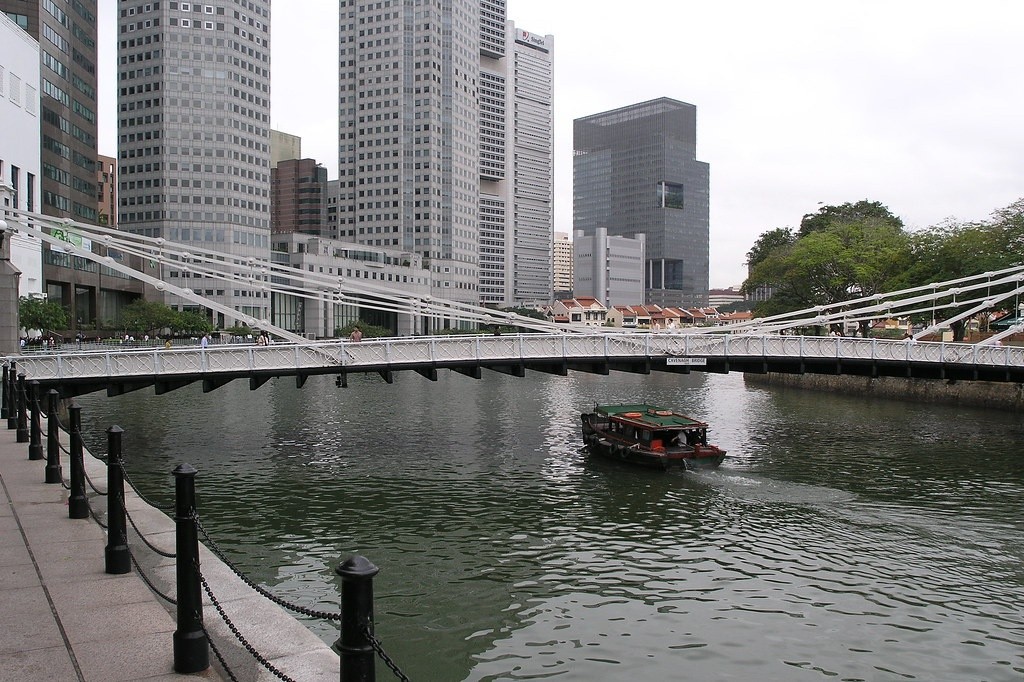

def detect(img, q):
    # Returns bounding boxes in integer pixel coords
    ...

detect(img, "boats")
[580,403,729,471]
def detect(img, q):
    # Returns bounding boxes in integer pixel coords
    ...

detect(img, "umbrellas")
[993,317,1024,327]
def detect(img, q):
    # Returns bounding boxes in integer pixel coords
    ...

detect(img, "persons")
[200,333,209,348]
[671,429,687,447]
[666,318,675,333]
[144,334,150,340]
[257,330,269,345]
[21,338,26,346]
[350,326,363,342]
[494,325,501,336]
[165,338,171,349]
[125,334,135,341]
[42,337,48,351]
[829,330,841,336]
[652,319,660,334]
[48,336,54,351]
